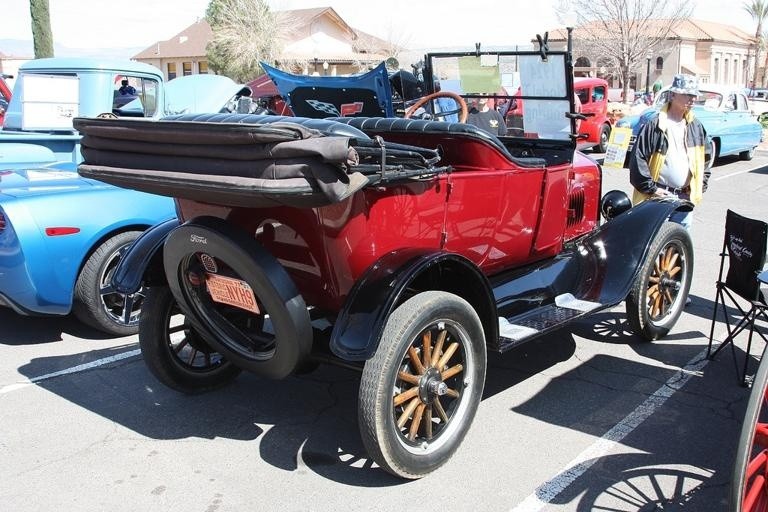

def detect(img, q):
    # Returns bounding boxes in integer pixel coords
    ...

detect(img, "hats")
[666,74,703,99]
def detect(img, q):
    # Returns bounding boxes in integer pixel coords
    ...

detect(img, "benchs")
[324,117,547,170]
[159,114,373,142]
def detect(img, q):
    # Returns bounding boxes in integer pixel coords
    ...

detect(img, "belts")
[655,182,690,195]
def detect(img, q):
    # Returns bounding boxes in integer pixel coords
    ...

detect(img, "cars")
[609,80,765,170]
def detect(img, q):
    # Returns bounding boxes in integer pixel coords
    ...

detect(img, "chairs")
[705,207,768,386]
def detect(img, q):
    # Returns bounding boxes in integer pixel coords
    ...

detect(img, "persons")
[119,80,133,95]
[630,74,713,305]
[653,80,663,98]
[466,86,510,136]
